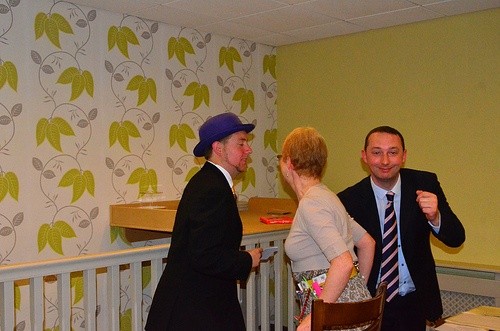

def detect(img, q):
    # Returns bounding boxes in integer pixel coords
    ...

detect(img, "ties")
[380,191,400,303]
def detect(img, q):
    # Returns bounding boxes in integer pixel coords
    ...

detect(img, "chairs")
[311,282,388,331]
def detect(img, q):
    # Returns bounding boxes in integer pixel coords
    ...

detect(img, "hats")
[193,112,255,157]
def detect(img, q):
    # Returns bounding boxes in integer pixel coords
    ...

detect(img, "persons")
[336,126,466,331]
[144,111,264,331]
[275,127,375,331]
